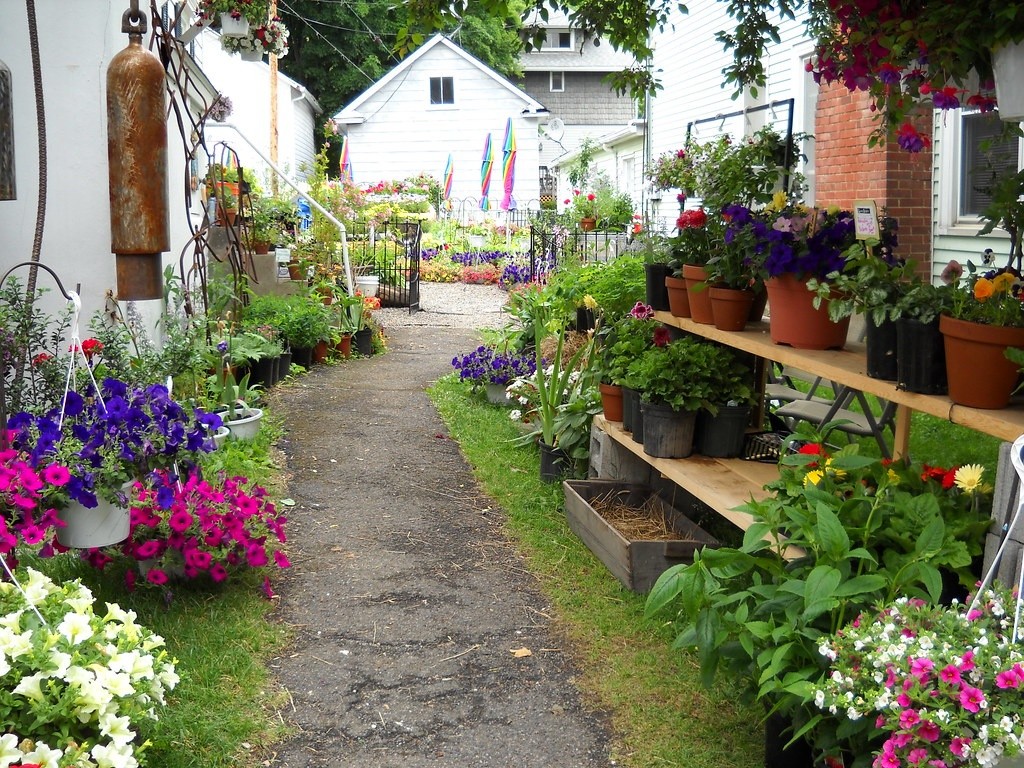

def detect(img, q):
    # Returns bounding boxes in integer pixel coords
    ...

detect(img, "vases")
[866,309,897,382]
[255,241,271,255]
[940,307,1024,409]
[356,274,380,296]
[600,384,622,421]
[641,399,698,458]
[201,425,230,450]
[53,476,136,548]
[683,262,724,325]
[709,283,755,332]
[763,701,816,768]
[240,47,262,61]
[287,261,310,280]
[621,385,632,431]
[336,333,352,359]
[220,12,249,37]
[763,273,856,351]
[580,219,595,231]
[218,408,263,441]
[272,357,278,383]
[138,559,201,582]
[211,389,260,413]
[218,209,237,228]
[749,288,768,324]
[751,165,795,194]
[217,181,239,197]
[485,384,512,403]
[355,329,372,355]
[896,311,947,394]
[318,288,332,305]
[631,391,644,444]
[244,357,271,388]
[700,403,751,457]
[315,340,328,362]
[644,263,678,311]
[208,366,237,383]
[665,276,691,318]
[292,345,313,368]
[280,353,293,377]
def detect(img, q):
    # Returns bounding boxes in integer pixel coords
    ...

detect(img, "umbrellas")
[444,154,453,199]
[499,117,518,212]
[479,133,494,214]
[339,136,354,182]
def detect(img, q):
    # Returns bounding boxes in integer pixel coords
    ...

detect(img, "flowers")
[805,1,1024,155]
[452,344,584,426]
[584,294,764,417]
[191,0,641,308]
[644,124,1024,397]
[0,262,392,768]
[641,419,1024,768]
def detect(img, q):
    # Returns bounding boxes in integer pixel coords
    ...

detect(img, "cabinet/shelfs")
[593,309,1024,563]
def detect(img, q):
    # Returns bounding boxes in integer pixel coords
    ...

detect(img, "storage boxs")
[562,479,719,595]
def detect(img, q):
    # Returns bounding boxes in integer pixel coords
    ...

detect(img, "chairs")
[765,359,911,470]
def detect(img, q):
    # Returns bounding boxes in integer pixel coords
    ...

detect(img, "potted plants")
[505,310,595,484]
